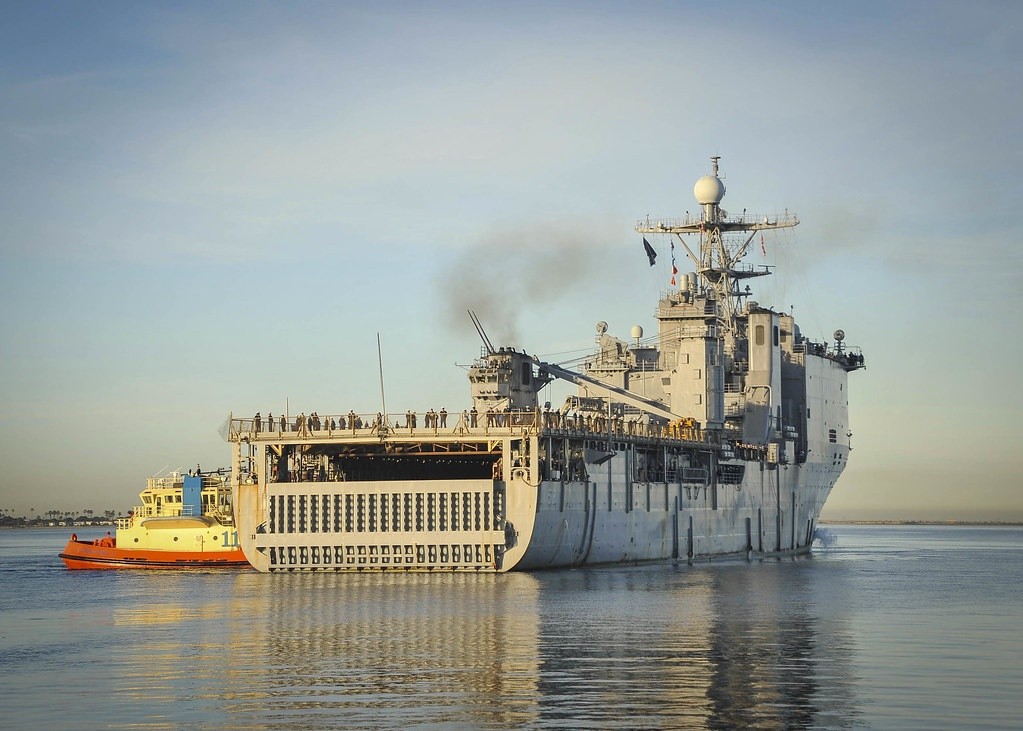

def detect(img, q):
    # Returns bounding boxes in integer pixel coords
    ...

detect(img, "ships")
[223,153,867,575]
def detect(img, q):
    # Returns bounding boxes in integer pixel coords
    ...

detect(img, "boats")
[57,462,253,571]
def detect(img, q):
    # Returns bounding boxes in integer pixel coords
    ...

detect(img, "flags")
[643,237,657,267]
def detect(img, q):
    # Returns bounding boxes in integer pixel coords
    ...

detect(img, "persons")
[544,407,670,436]
[254,406,542,433]
